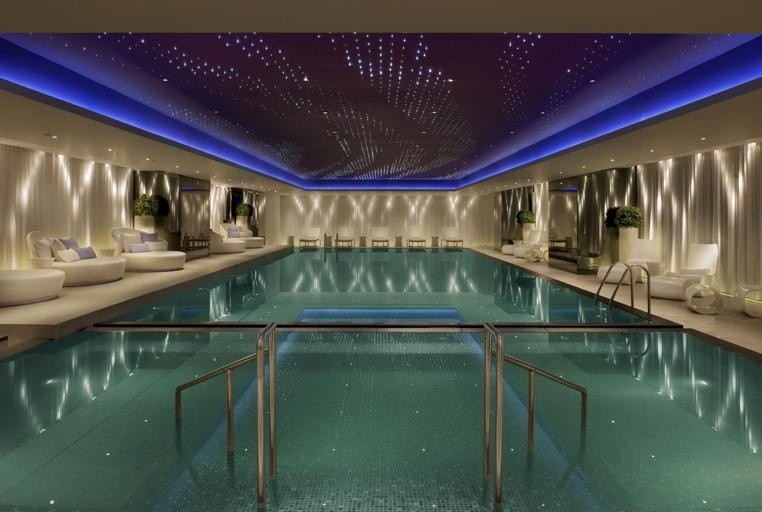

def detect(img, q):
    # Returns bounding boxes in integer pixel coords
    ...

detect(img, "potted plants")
[133,195,160,234]
[515,211,536,241]
[614,205,642,262]
[236,203,250,225]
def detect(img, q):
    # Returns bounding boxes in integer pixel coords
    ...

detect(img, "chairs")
[298,226,465,251]
[502,230,720,303]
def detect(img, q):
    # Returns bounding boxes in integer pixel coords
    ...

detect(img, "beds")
[208,225,244,255]
[111,225,186,272]
[0,268,65,307]
[26,230,126,286]
[219,223,264,249]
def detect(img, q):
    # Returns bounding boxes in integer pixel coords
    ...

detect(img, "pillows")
[123,229,164,253]
[34,236,98,263]
[225,225,247,237]
